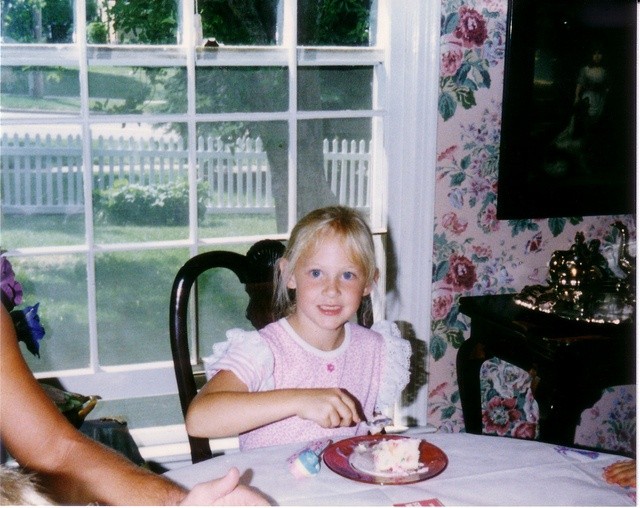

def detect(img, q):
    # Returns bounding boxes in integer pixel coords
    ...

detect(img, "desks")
[458,289,636,447]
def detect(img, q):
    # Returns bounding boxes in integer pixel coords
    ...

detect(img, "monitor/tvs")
[495,0,637,221]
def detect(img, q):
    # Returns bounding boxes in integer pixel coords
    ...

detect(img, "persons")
[0,299,273,507]
[604,460,635,489]
[186,206,412,452]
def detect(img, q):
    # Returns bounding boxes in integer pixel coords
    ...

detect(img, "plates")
[323,435,448,485]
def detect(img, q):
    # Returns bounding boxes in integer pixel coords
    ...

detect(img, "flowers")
[1,251,48,360]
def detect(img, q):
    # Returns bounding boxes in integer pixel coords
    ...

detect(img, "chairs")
[168,238,379,458]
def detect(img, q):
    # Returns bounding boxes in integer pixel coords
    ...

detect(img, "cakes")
[355,438,424,474]
